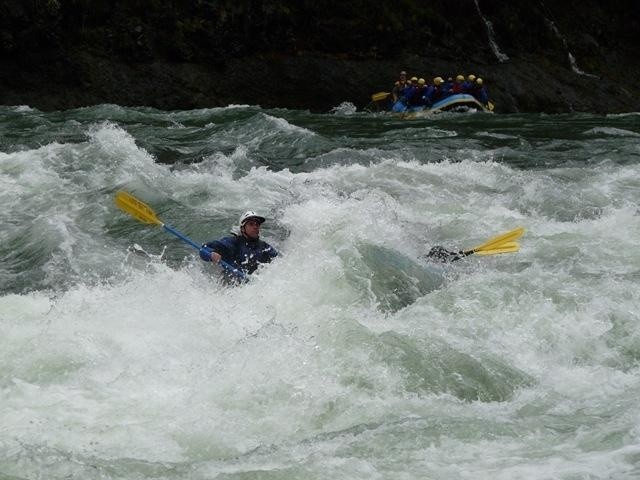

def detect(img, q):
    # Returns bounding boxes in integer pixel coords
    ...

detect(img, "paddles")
[449,227,525,262]
[116,191,250,280]
[372,91,392,101]
[451,241,520,255]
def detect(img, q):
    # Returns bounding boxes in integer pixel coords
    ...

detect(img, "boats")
[364,91,489,116]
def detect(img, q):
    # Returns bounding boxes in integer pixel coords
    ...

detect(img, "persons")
[199,211,278,286]
[392,70,488,113]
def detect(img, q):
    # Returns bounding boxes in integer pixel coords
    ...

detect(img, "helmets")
[400,71,407,75]
[456,75,464,81]
[476,78,483,85]
[239,210,265,229]
[434,77,445,86]
[418,78,425,85]
[410,77,418,82]
[468,74,476,82]
